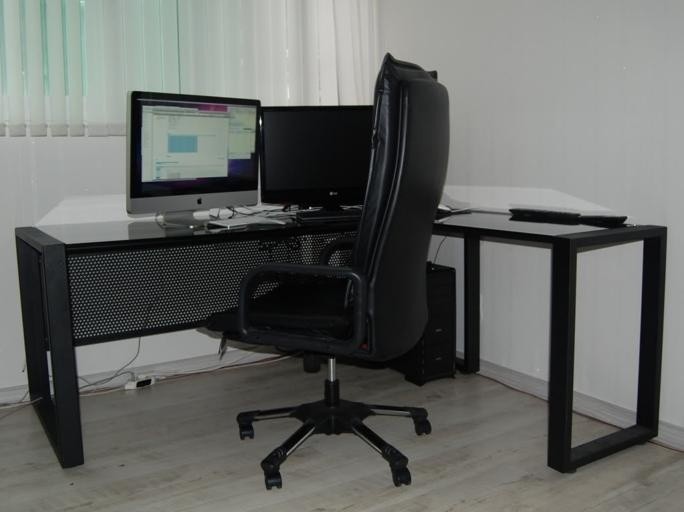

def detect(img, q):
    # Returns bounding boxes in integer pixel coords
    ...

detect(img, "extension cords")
[124,377,155,390]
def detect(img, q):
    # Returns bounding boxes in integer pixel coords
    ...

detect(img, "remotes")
[509,206,580,220]
[579,216,628,227]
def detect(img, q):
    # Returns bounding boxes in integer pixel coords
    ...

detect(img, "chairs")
[204,51,452,489]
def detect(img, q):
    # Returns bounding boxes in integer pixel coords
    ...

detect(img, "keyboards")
[296,210,360,220]
[208,216,265,228]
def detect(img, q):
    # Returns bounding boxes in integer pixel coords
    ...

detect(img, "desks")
[14,201,669,474]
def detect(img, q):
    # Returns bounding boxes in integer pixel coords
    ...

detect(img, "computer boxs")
[389,261,457,387]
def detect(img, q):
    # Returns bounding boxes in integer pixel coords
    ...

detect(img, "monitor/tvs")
[260,105,375,221]
[126,90,262,229]
